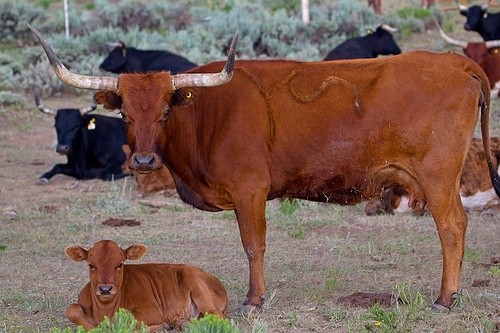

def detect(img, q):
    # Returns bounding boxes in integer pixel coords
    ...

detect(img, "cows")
[431,16,500,98]
[454,0,500,41]
[98,39,199,75]
[26,21,500,315]
[322,23,402,62]
[33,93,176,199]
[64,239,230,333]
[365,136,500,214]
